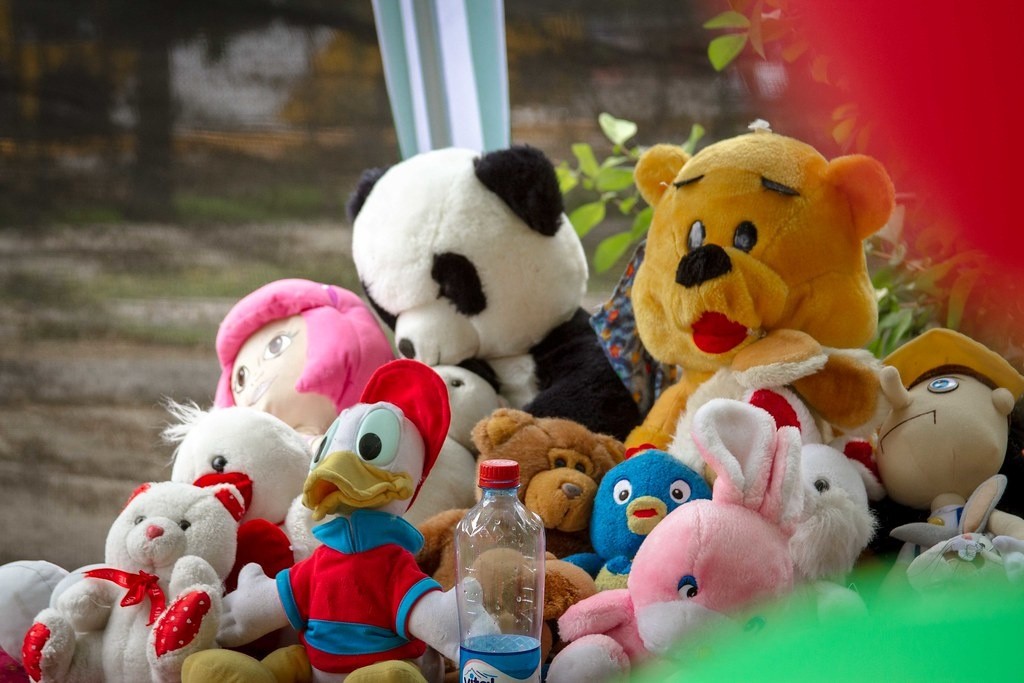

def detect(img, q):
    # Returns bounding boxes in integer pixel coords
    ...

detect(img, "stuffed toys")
[0,329,1024,683]
[349,145,640,439]
[217,280,399,439]
[625,122,893,449]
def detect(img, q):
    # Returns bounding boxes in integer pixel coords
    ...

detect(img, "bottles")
[454,459,546,683]
